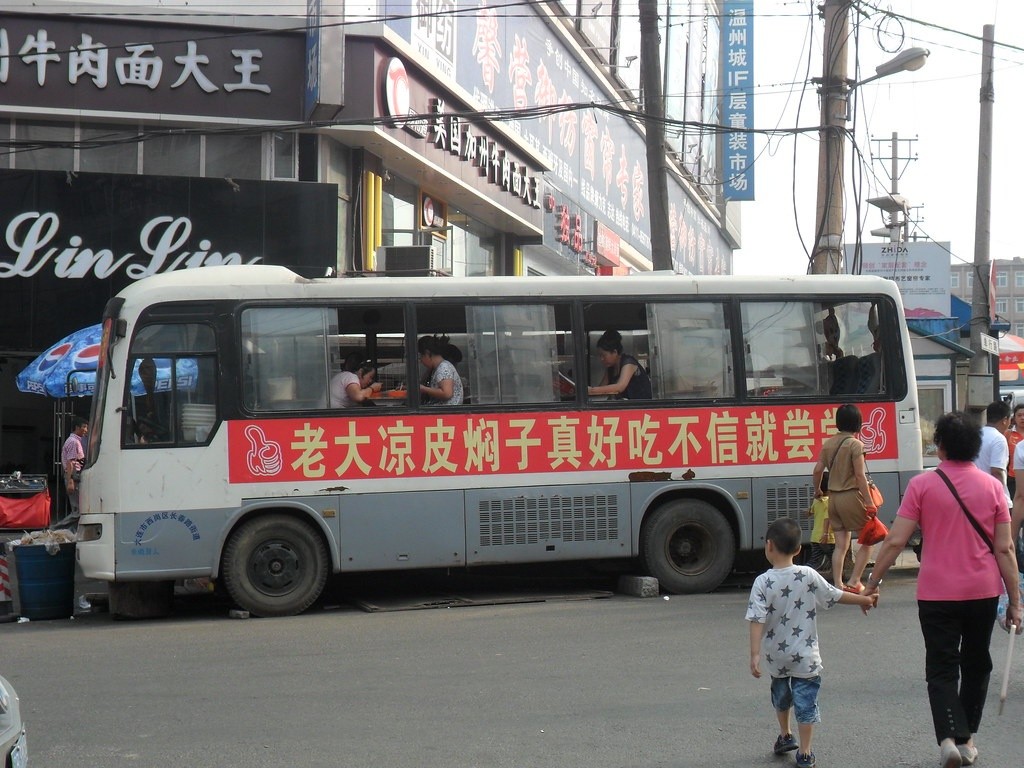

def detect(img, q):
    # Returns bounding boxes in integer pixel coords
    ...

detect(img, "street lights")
[865,191,910,242]
[811,46,928,274]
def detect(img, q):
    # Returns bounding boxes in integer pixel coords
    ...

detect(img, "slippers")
[841,583,864,594]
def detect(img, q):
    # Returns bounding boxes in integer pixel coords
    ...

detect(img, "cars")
[1000,389,1024,418]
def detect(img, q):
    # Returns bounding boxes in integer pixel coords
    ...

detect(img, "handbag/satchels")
[858,478,884,509]
[856,506,890,546]
[997,573,1024,635]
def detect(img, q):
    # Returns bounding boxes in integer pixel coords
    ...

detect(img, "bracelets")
[866,573,883,587]
[591,385,595,394]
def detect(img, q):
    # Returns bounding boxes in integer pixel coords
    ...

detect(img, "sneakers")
[796,748,816,768]
[773,733,799,755]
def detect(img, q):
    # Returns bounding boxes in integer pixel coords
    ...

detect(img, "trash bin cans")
[8,528,78,622]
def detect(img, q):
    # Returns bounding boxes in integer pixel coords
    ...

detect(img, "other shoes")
[955,744,977,765]
[940,741,962,768]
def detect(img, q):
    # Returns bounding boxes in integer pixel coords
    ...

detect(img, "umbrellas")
[999,332,1024,381]
[15,322,267,445]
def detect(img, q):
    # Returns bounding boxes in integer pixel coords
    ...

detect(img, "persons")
[50,416,88,531]
[859,410,1024,768]
[315,325,653,408]
[812,404,879,597]
[745,518,879,768]
[976,400,1024,576]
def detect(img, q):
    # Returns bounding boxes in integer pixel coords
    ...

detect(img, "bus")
[64,264,925,619]
